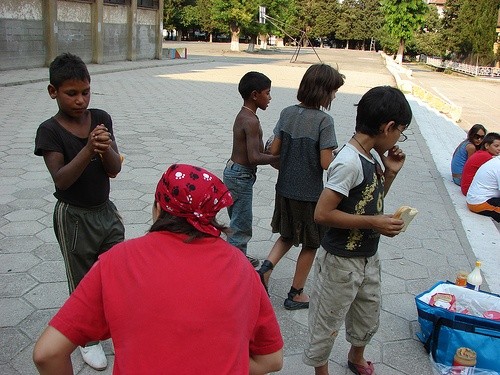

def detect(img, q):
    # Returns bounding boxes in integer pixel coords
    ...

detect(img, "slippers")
[347,359,377,375]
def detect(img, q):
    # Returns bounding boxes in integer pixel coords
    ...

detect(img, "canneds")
[453,347,477,367]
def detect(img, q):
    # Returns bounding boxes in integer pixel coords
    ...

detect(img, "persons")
[300,86,413,375]
[451,123,487,186]
[466,154,500,223]
[32,165,284,375]
[34,52,126,370]
[222,72,281,265]
[461,133,500,195]
[253,63,345,307]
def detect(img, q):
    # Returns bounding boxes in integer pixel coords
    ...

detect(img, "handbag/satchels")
[413,278,500,374]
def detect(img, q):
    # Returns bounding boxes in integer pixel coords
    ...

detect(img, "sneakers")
[247,255,257,265]
[78,341,108,371]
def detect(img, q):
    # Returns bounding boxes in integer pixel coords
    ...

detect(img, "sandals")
[284,286,311,310]
[255,260,275,298]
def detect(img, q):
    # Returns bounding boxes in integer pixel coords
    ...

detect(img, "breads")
[393,206,418,232]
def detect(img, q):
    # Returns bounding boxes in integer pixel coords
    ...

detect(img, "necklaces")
[352,134,386,177]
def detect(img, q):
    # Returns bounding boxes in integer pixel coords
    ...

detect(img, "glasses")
[393,125,408,143]
[473,133,485,140]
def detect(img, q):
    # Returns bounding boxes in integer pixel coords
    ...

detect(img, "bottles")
[466,261,482,291]
